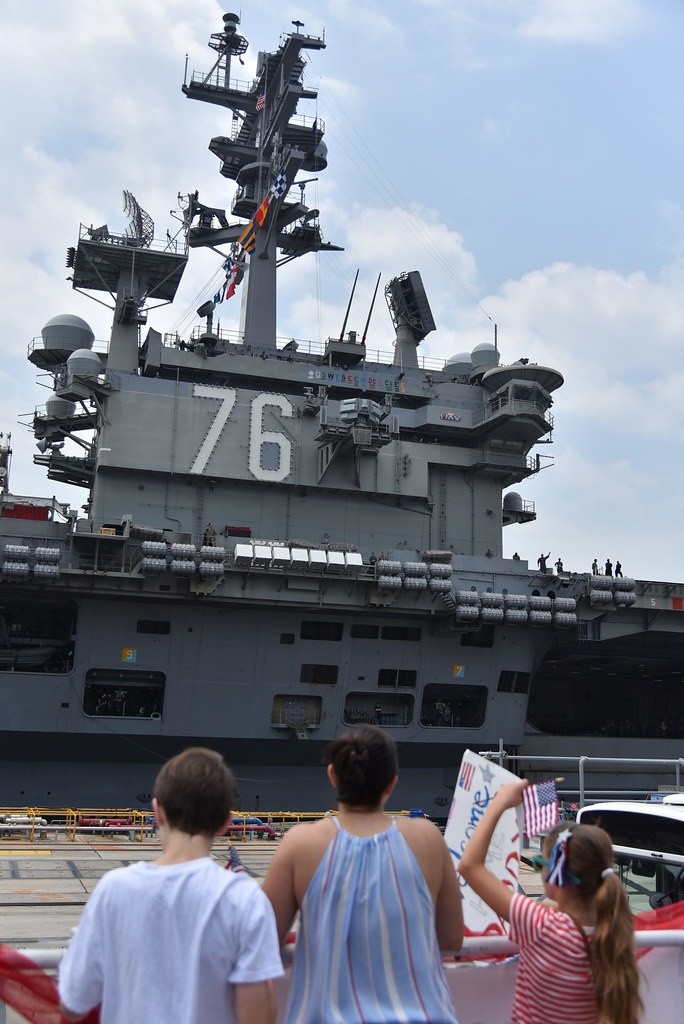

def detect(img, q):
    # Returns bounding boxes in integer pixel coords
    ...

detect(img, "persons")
[260,725,467,1023]
[180,340,186,350]
[59,749,288,1024]
[537,552,551,574]
[457,780,647,1024]
[485,549,493,557]
[555,557,563,574]
[615,561,623,577]
[605,559,613,576]
[592,558,600,576]
[513,552,520,560]
[203,522,218,546]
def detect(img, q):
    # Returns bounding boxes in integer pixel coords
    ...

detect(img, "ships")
[1,10,684,808]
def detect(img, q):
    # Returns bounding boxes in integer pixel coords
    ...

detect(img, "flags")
[443,749,522,964]
[254,168,293,228]
[520,781,558,840]
[238,223,256,255]
[213,257,241,304]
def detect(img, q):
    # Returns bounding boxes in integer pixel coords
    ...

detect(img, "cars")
[575,792,684,869]
